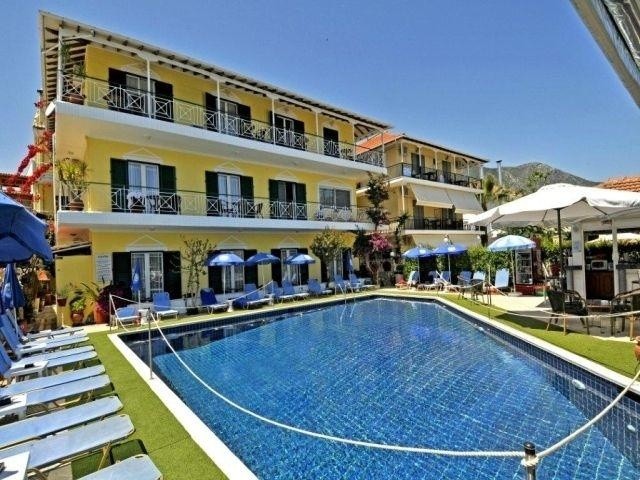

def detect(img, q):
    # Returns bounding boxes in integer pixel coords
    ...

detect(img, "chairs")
[249,203,264,219]
[130,196,145,213]
[545,285,592,335]
[0,292,178,480]
[396,269,510,293]
[163,195,181,215]
[423,219,433,229]
[219,199,237,217]
[199,273,376,313]
[610,287,640,341]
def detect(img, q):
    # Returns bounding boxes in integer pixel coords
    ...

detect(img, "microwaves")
[591,260,608,270]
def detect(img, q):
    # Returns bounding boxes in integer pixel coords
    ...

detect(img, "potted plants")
[394,265,404,283]
[66,61,89,105]
[309,225,348,294]
[103,95,123,111]
[80,277,114,323]
[56,288,68,306]
[69,280,87,326]
[54,156,94,211]
[169,238,218,315]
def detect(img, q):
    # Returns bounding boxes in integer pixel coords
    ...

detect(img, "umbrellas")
[0,191,57,268]
[206,252,245,294]
[0,263,28,339]
[284,252,316,285]
[241,252,281,293]
[130,258,145,308]
[465,182,640,291]
[482,234,537,292]
[402,245,434,284]
[431,242,468,283]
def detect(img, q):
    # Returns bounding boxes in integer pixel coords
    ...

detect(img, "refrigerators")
[514,237,541,295]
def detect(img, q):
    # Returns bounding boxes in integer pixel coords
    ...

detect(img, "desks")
[455,180,467,185]
[146,195,160,213]
[587,300,614,335]
